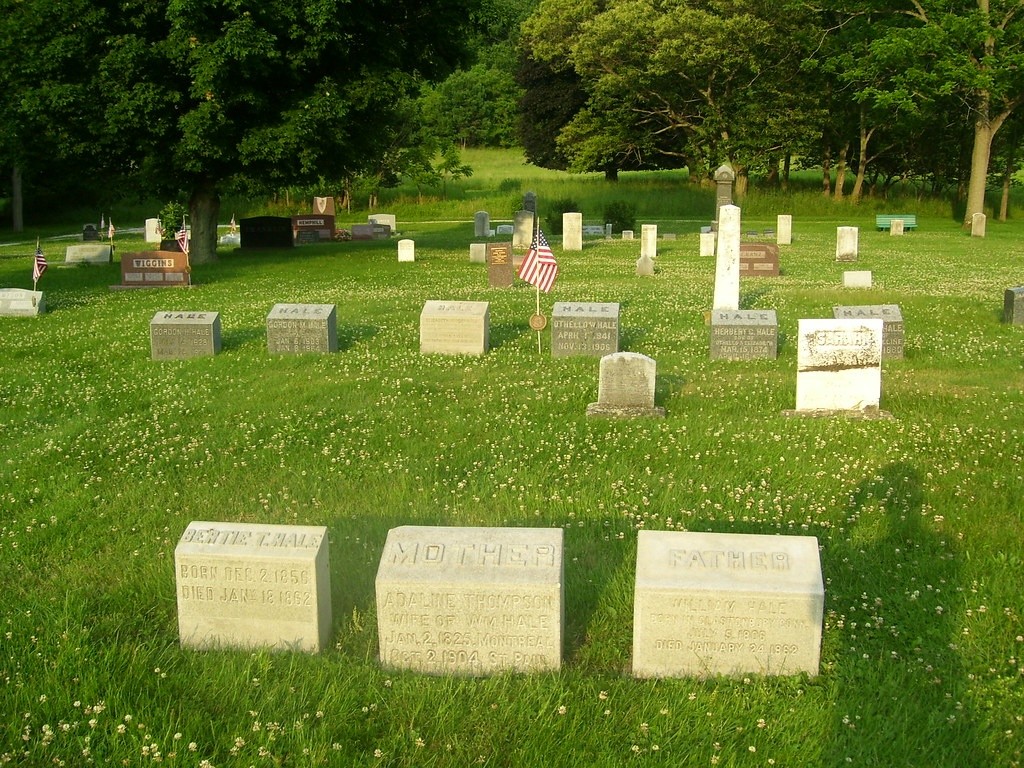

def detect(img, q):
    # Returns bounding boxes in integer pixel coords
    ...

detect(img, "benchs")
[876,215,918,232]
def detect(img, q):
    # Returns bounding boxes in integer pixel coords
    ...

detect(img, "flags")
[100,217,105,228]
[32,248,47,282]
[518,229,558,293]
[108,224,115,238]
[231,218,236,231]
[155,223,161,235]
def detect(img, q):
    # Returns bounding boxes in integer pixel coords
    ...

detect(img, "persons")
[176,226,189,254]
